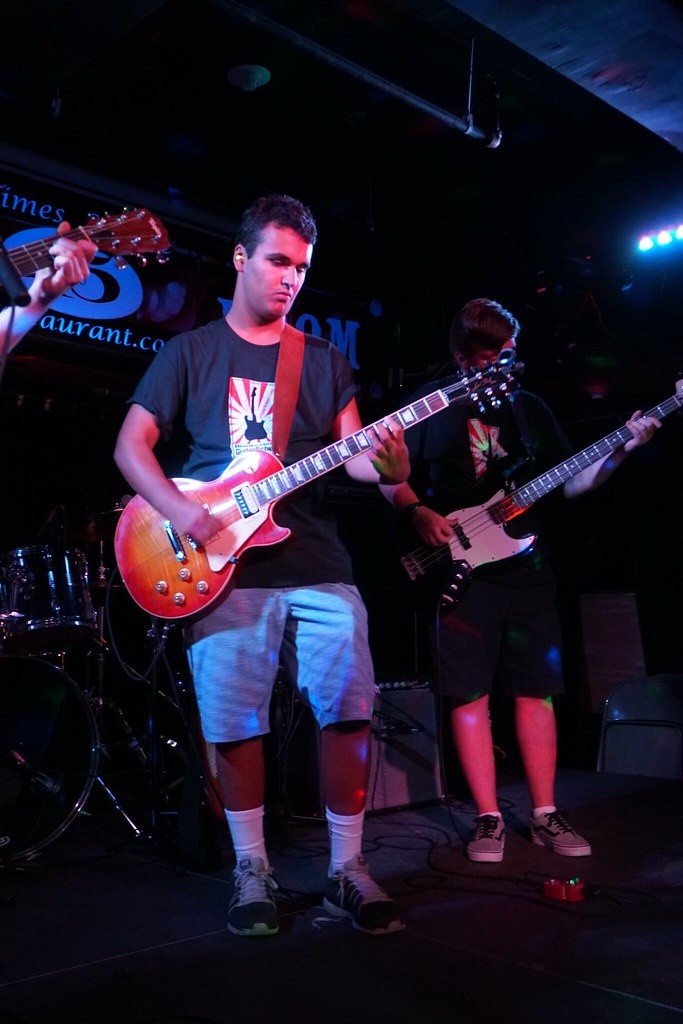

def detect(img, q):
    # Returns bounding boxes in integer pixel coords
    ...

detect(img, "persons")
[377,296,662,863]
[113,201,412,939]
[0,217,100,370]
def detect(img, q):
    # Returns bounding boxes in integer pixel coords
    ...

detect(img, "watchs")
[406,502,423,520]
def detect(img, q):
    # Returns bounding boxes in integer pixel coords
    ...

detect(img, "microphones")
[39,504,63,536]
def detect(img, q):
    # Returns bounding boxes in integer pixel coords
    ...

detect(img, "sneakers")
[225,857,281,935]
[468,814,505,862]
[530,810,591,857]
[313,856,407,935]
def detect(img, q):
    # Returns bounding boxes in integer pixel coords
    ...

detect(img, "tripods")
[69,502,209,845]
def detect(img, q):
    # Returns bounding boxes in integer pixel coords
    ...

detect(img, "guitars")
[399,376,683,617]
[0,204,171,287]
[114,359,521,623]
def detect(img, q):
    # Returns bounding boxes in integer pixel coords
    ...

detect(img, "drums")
[0,544,98,657]
[0,650,102,866]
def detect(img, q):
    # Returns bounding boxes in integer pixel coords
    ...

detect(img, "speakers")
[313,676,448,818]
[323,475,420,684]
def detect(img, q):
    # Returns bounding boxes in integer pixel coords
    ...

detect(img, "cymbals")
[96,506,123,515]
[65,582,125,590]
